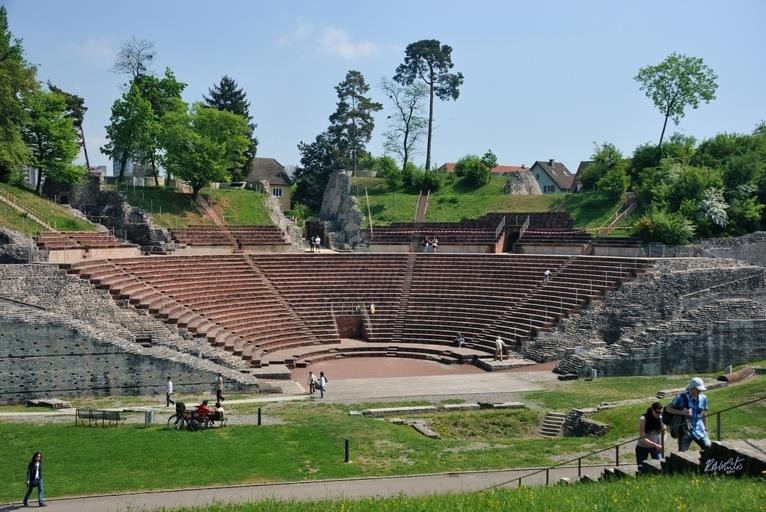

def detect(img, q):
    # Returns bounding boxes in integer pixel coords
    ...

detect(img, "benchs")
[74,407,125,428]
[183,410,227,429]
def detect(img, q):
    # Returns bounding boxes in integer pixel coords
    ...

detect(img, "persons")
[317,370,328,399]
[214,372,225,403]
[197,400,214,428]
[163,376,176,408]
[492,335,505,362]
[308,235,314,252]
[452,332,460,346]
[311,234,321,253]
[368,301,375,315]
[665,375,714,453]
[22,450,49,507]
[457,333,465,349]
[211,402,225,426]
[428,235,439,253]
[630,401,668,466]
[544,268,552,284]
[420,235,431,253]
[307,370,318,396]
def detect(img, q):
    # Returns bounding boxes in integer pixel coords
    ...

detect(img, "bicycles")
[168,409,207,431]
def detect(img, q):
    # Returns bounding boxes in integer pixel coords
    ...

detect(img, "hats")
[692,377,706,390]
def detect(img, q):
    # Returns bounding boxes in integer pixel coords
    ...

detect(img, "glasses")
[654,409,663,414]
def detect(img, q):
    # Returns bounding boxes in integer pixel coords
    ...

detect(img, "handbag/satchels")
[662,393,690,430]
[30,481,39,486]
